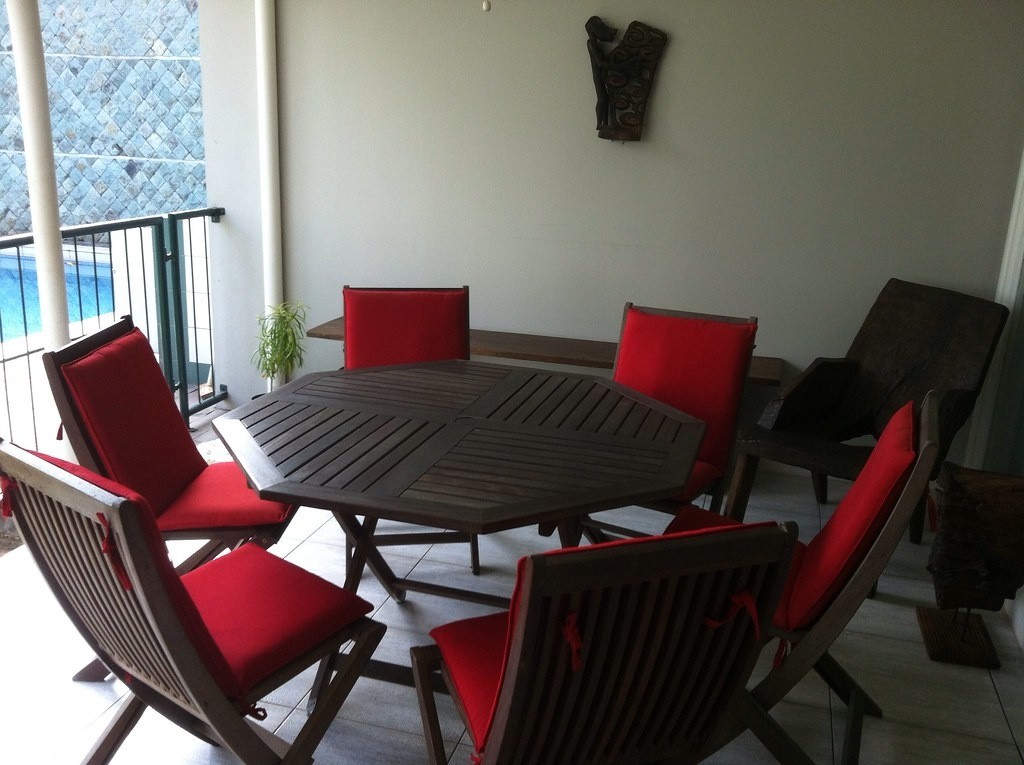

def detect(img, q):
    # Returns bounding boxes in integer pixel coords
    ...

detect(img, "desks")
[305,316,787,491]
[210,359,706,717]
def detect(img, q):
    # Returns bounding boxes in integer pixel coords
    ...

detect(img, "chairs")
[43,315,299,555]
[0,438,389,765]
[723,275,1011,546]
[410,520,799,765]
[584,304,760,542]
[665,389,941,764]
[341,287,481,576]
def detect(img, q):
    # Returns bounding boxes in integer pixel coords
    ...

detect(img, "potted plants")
[251,300,310,402]
